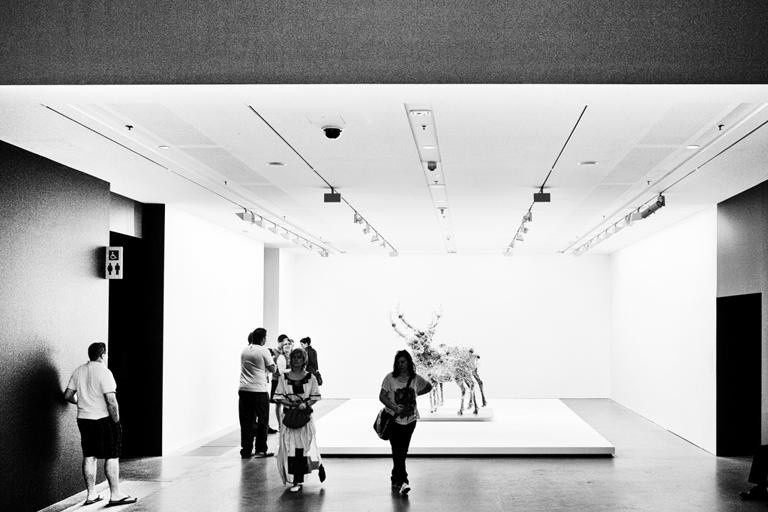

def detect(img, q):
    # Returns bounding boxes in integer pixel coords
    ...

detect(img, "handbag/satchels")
[282,407,310,429]
[373,408,395,440]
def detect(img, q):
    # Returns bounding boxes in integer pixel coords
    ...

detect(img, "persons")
[238,328,326,492]
[64,343,137,505]
[380,350,432,494]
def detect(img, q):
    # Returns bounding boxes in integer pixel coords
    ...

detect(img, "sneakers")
[392,481,411,495]
[242,450,275,459]
[268,428,277,434]
[289,484,302,492]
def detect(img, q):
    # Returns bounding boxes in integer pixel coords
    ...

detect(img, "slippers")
[109,495,137,505]
[83,495,103,505]
